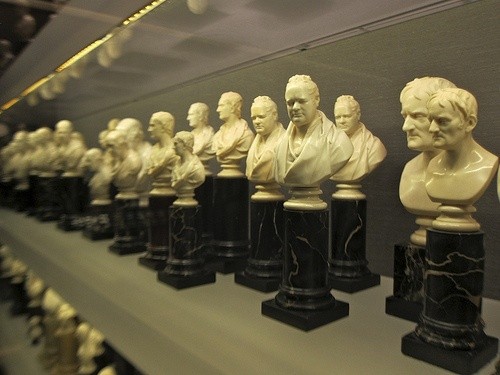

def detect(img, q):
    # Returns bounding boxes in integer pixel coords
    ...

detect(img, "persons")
[211,91,255,164]
[245,95,289,185]
[144,110,176,180]
[399,76,462,218]
[170,131,206,193]
[327,95,388,184]
[424,87,500,207]
[0,118,152,193]
[0,244,117,375]
[186,102,217,163]
[272,74,354,188]
